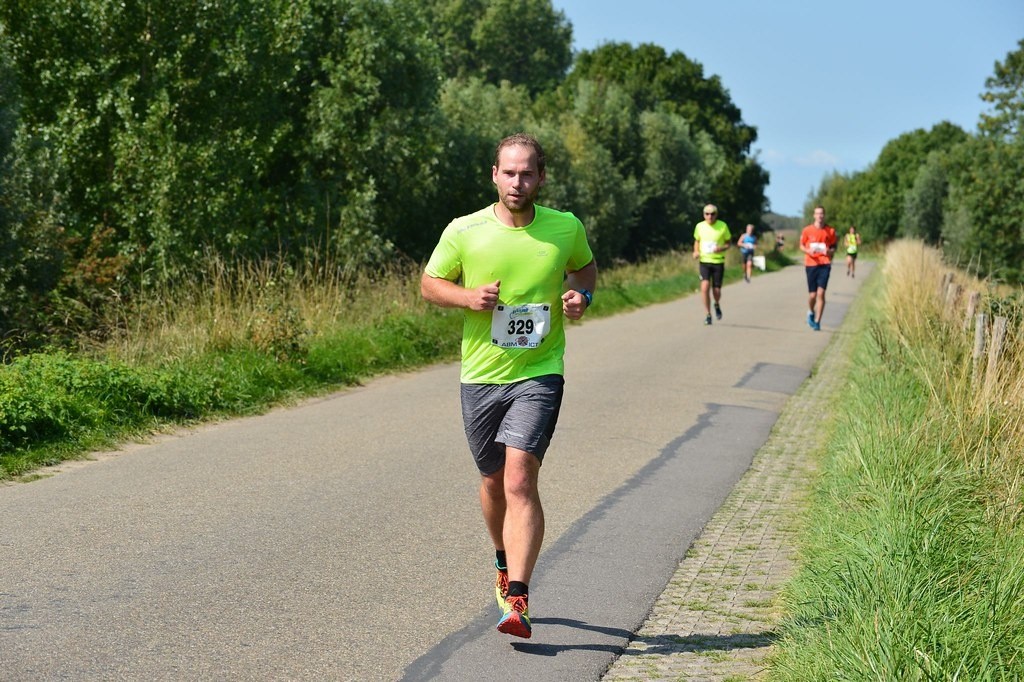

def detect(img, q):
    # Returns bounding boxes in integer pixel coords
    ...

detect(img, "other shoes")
[814,323,820,330]
[808,309,815,327]
[706,317,711,323]
[715,304,721,318]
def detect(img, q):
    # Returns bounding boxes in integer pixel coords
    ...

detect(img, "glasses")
[706,213,714,215]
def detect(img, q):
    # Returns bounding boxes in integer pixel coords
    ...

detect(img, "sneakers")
[497,589,531,638]
[495,563,509,616]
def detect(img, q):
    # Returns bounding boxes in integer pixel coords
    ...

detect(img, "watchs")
[579,289,592,307]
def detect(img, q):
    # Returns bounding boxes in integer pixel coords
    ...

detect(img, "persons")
[737,224,759,283]
[776,233,784,252]
[692,204,732,325]
[844,226,861,279]
[421,133,598,638]
[800,205,837,331]
